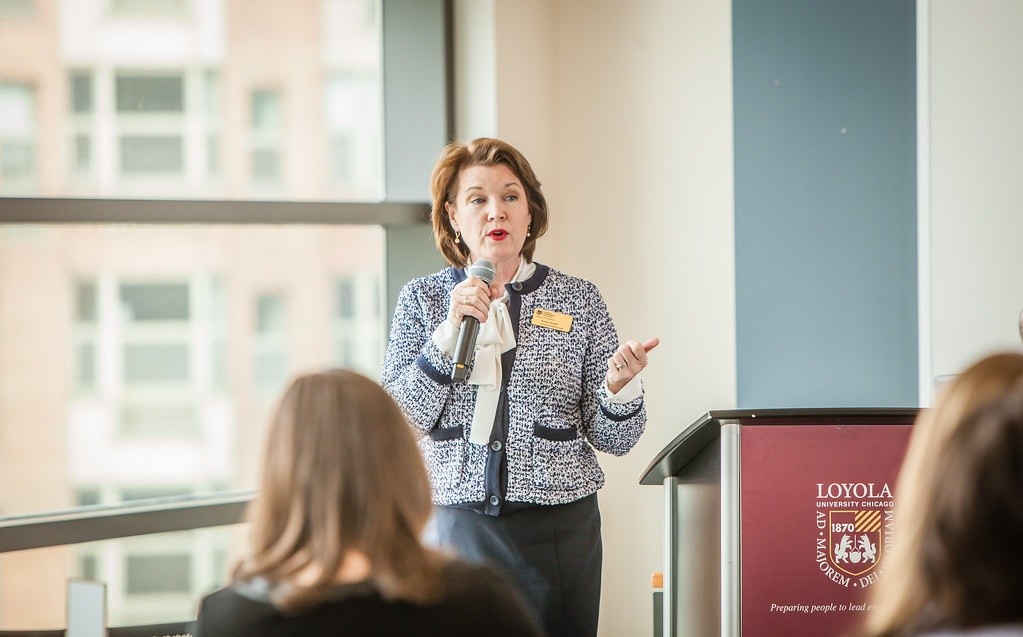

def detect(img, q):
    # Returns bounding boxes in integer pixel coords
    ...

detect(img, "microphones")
[450,260,495,381]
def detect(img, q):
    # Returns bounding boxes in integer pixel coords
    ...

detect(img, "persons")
[381,135,660,637]
[855,351,1023,636]
[191,367,543,636]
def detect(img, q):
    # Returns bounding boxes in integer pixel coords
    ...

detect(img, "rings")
[616,361,627,370]
[463,296,470,306]
